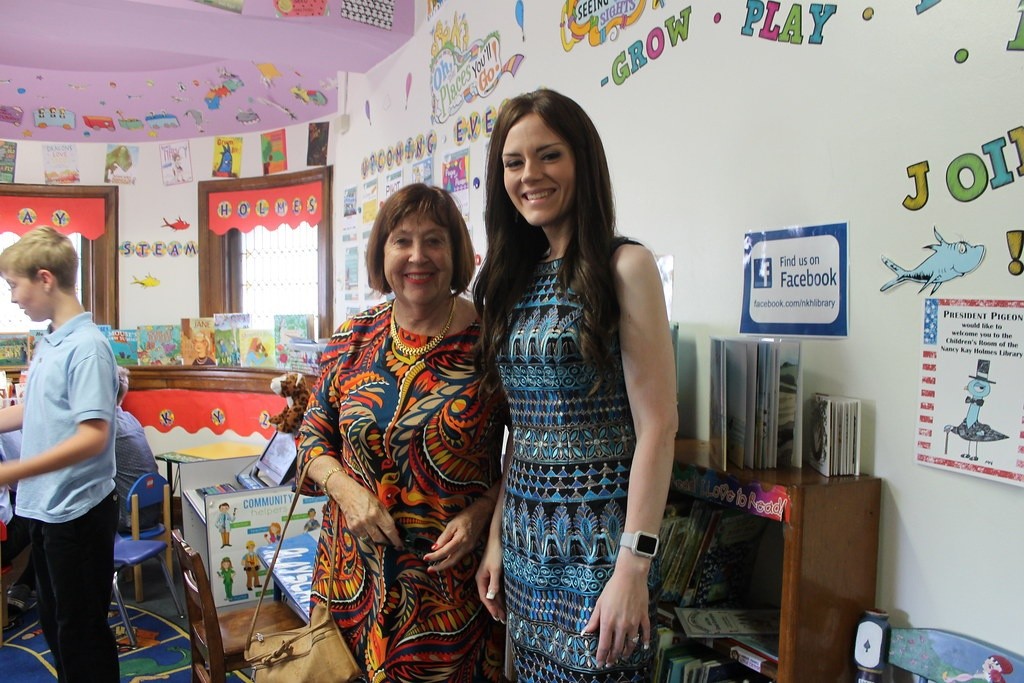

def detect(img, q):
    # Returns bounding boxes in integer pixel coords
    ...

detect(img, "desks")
[257,533,317,625]
[155,452,205,509]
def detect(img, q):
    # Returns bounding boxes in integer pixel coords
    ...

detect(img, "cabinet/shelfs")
[180,455,330,607]
[657,438,880,683]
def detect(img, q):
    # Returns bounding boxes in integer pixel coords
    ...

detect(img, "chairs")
[853,608,1024,683]
[119,473,173,603]
[172,529,307,683]
[110,532,185,649]
[0,520,13,626]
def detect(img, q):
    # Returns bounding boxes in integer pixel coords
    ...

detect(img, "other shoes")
[7,581,28,610]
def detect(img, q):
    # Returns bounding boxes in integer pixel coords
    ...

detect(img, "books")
[652,500,780,683]
[670,321,861,476]
[1,312,332,410]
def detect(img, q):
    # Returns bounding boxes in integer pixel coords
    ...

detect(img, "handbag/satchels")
[244,603,362,683]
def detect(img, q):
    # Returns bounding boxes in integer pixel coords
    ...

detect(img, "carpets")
[0,598,253,683]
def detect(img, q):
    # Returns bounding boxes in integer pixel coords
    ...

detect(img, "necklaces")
[391,296,457,355]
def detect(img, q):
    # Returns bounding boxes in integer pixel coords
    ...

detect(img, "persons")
[0,365,161,631]
[291,184,514,683]
[472,88,678,683]
[0,226,120,683]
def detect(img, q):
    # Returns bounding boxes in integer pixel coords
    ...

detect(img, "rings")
[359,535,370,543]
[627,634,639,646]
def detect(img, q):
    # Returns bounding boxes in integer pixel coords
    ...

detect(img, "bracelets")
[322,467,346,500]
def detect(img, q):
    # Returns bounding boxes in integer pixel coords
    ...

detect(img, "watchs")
[620,531,660,559]
